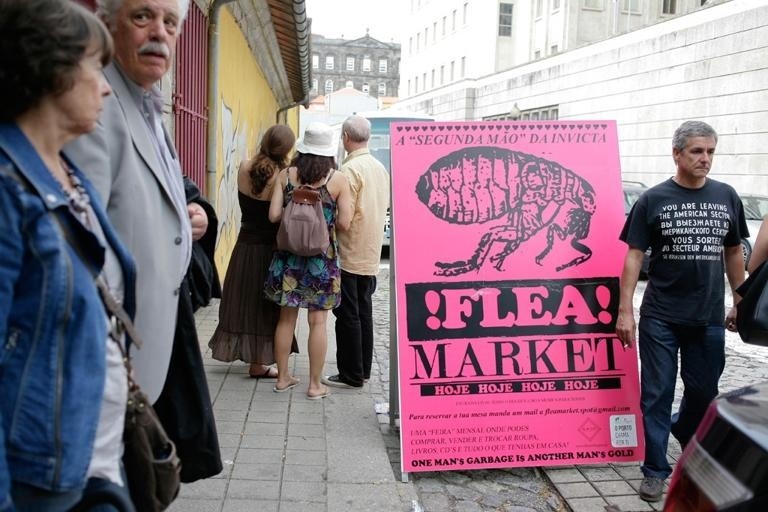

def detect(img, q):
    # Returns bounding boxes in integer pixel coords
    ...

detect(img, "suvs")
[621,178,650,275]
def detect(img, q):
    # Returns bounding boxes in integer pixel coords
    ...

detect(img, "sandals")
[249,365,280,379]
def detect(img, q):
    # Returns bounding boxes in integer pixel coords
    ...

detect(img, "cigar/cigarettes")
[623,344,630,348]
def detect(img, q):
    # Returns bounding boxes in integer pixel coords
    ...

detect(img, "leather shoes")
[640,475,666,502]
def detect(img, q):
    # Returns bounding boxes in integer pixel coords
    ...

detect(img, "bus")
[336,111,440,256]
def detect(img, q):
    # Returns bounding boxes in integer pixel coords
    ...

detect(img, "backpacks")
[274,183,332,255]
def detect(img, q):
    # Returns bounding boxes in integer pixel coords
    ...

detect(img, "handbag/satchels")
[126,379,181,511]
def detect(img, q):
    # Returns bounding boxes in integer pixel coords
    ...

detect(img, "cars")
[735,193,768,270]
[663,379,768,510]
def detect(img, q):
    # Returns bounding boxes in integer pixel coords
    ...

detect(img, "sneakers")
[322,373,363,390]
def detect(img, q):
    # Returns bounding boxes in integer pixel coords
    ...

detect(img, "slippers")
[272,376,303,393]
[307,386,333,401]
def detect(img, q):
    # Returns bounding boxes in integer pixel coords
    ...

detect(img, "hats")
[294,123,337,158]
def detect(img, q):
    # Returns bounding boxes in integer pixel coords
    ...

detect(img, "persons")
[267,127,355,399]
[747,214,768,277]
[613,120,752,503]
[207,126,298,379]
[2,2,159,511]
[57,2,214,511]
[321,112,391,389]
